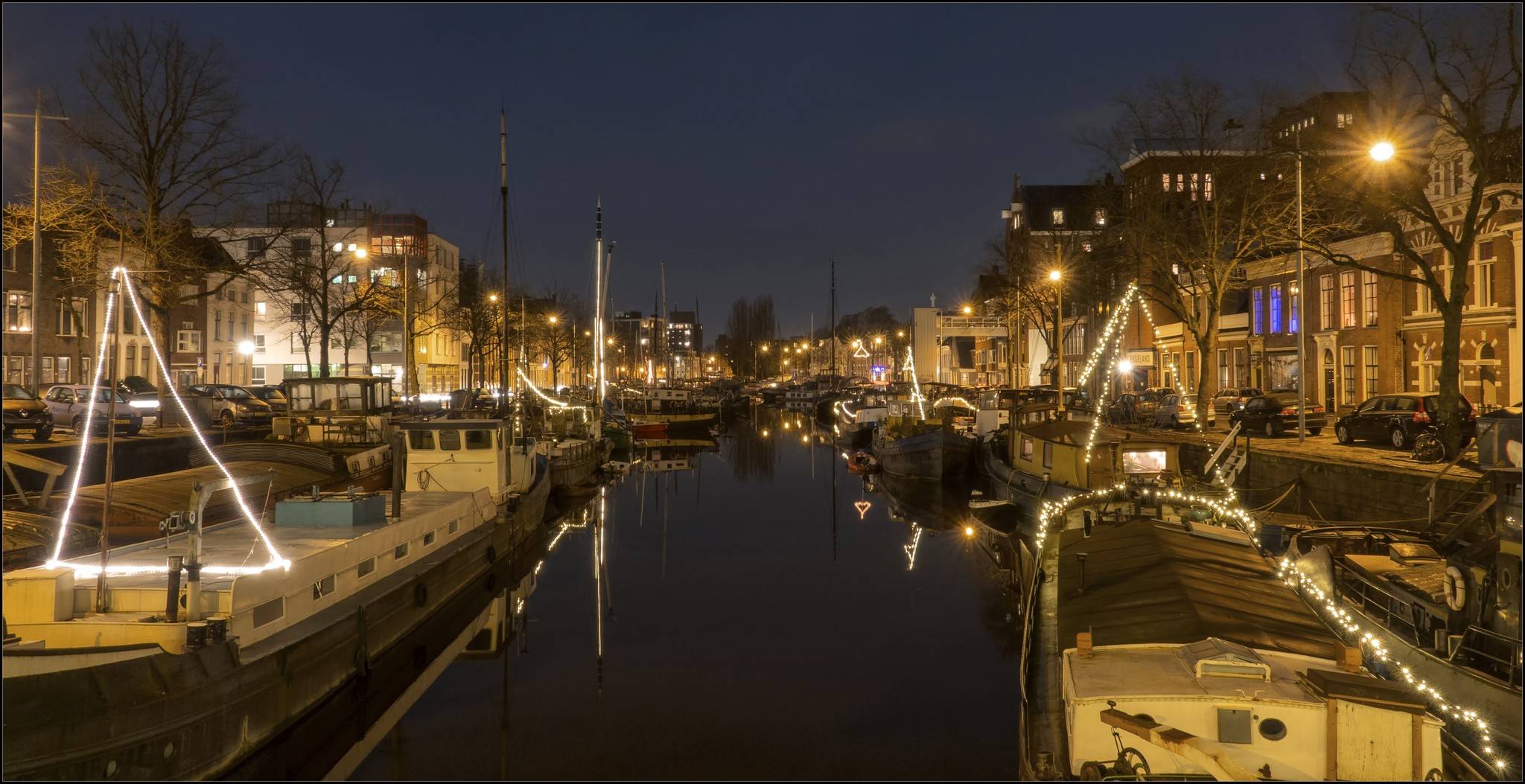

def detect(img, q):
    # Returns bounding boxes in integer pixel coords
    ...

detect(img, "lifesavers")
[884,427,889,436]
[670,400,678,408]
[679,400,690,408]
[1442,566,1466,612]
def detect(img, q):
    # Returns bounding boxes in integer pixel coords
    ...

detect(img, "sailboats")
[0,117,1525,784]
